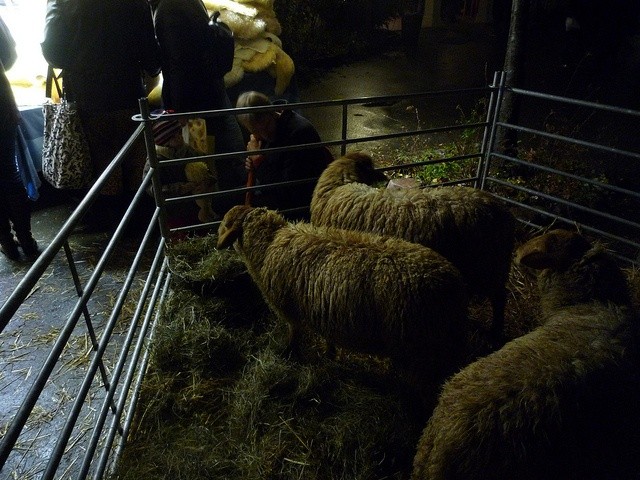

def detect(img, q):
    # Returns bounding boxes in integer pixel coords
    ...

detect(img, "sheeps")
[216,203,496,360]
[308,152,534,259]
[412,228,640,480]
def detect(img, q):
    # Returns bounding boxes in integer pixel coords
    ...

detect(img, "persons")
[233,89,335,214]
[155,1,235,151]
[0,18,42,263]
[144,110,217,234]
[41,0,155,232]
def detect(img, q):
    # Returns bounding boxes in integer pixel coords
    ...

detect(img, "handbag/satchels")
[40,61,93,190]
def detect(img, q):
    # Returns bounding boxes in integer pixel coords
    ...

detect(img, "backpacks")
[196,2,234,76]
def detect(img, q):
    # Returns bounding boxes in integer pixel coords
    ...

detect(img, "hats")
[149,109,185,145]
